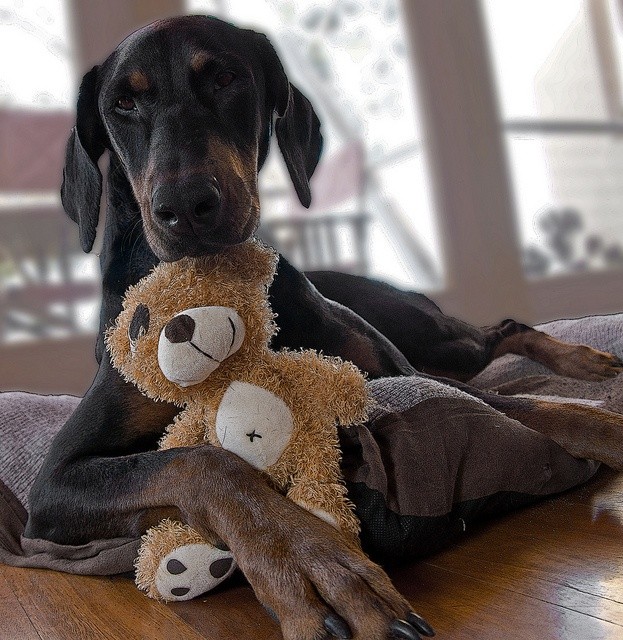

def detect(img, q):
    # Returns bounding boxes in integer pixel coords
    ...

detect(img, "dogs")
[25,14,623,640]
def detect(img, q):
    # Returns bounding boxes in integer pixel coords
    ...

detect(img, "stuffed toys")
[102,236,377,604]
